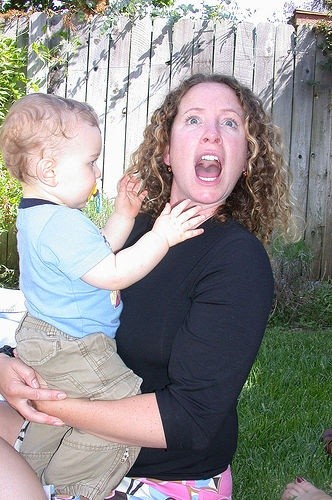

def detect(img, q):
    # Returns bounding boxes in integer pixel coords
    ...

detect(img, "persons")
[0,93,206,500]
[0,73,308,500]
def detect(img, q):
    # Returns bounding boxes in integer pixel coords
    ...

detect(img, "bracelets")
[0,344,17,358]
